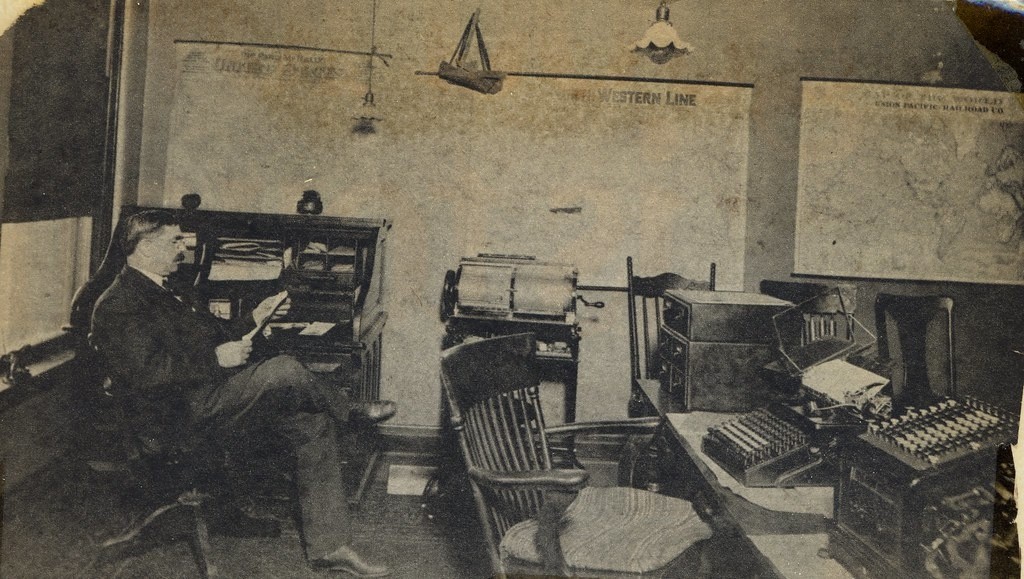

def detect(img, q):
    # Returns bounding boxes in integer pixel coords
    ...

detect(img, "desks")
[661,409,1024,577]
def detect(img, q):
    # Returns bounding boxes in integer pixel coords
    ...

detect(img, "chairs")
[627,256,716,415]
[441,331,717,579]
[874,293,956,408]
[63,326,287,576]
[762,279,857,348]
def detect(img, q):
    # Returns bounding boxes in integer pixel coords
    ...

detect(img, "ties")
[162,280,193,309]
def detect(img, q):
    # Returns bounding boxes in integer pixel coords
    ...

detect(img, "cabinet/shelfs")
[420,250,581,516]
[117,210,392,503]
[654,288,801,410]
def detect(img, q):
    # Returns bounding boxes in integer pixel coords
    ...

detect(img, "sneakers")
[347,399,397,431]
[309,545,390,579]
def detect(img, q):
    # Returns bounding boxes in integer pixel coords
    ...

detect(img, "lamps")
[627,1,694,60]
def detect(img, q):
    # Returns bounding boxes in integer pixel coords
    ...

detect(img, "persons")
[92,205,402,577]
